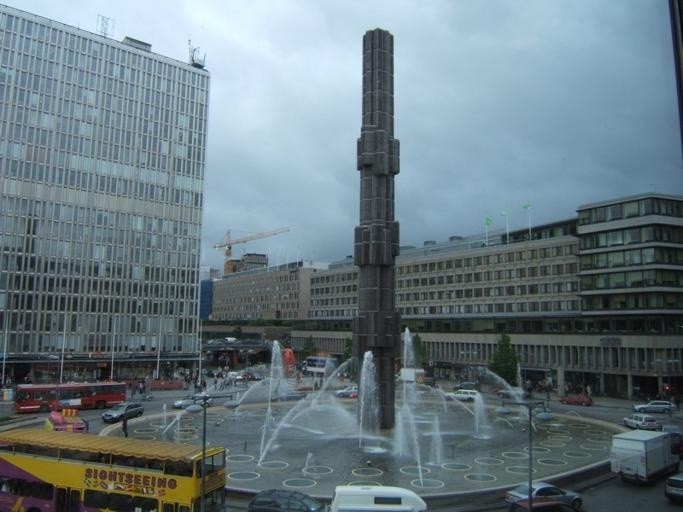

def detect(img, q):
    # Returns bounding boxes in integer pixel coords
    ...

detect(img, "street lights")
[495,398,553,511]
[186,393,239,512]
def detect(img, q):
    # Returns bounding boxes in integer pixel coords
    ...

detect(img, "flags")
[518,202,530,209]
[483,214,492,228]
[499,209,507,215]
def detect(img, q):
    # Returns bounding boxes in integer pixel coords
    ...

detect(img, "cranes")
[212,225,291,274]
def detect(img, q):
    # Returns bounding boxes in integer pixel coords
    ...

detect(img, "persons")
[523,377,602,400]
[0,350,230,395]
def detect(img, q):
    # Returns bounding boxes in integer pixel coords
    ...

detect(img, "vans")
[329,483,427,512]
[446,389,479,401]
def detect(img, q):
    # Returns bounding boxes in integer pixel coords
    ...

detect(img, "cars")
[174,392,211,409]
[498,385,529,400]
[666,474,683,504]
[227,369,263,382]
[504,479,583,512]
[102,402,144,423]
[283,391,305,400]
[660,423,682,445]
[561,392,592,407]
[336,384,357,398]
[208,336,241,344]
[456,383,481,392]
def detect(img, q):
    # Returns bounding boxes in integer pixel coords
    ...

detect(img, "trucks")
[394,367,425,382]
[610,431,680,482]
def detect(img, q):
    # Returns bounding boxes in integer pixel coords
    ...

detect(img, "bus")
[14,382,126,413]
[302,356,338,377]
[0,428,226,511]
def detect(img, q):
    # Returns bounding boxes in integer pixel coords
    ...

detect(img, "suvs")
[634,399,674,413]
[249,487,322,512]
[625,413,658,430]
